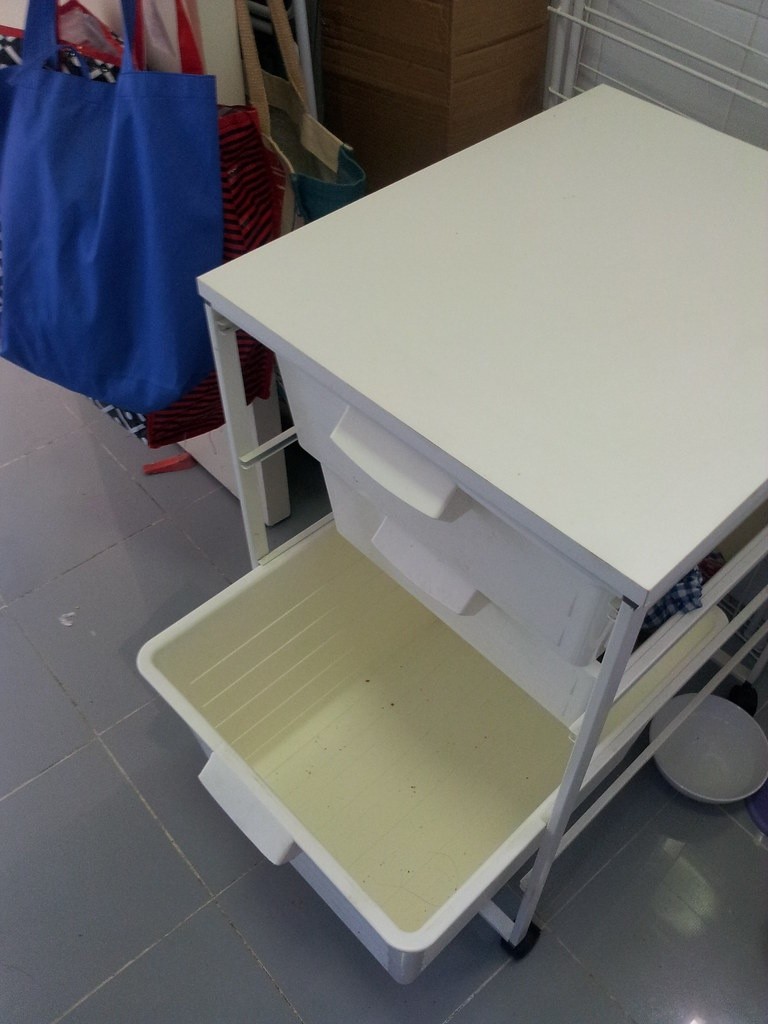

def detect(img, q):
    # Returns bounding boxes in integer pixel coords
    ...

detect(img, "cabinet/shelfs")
[135,82,768,986]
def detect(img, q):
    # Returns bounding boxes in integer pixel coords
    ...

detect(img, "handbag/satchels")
[0,1,370,447]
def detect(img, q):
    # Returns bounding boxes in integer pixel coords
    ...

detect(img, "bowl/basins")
[649,693,768,805]
